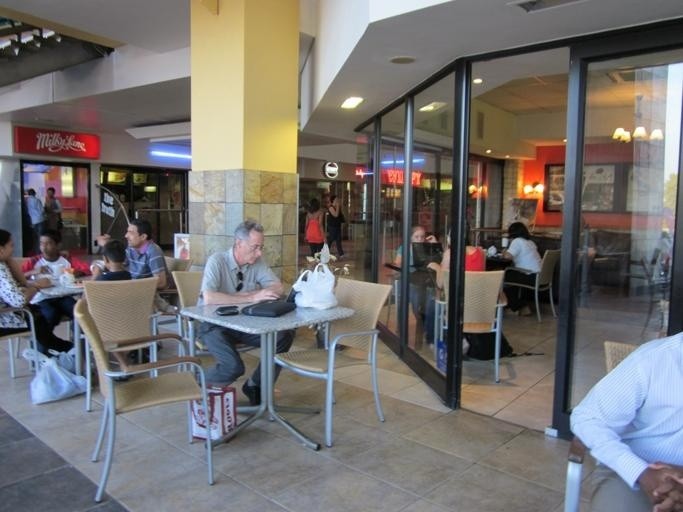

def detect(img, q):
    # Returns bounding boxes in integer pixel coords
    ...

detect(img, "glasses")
[245,244,266,251]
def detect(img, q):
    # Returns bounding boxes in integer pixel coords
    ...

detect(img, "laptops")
[412,242,444,272]
[262,268,307,302]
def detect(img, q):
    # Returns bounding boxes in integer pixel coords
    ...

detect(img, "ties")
[236,271,244,292]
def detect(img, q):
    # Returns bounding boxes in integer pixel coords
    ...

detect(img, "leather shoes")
[241,378,262,407]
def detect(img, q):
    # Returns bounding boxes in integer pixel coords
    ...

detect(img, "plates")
[33,266,90,289]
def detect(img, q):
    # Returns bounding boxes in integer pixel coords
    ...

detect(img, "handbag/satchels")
[190,381,237,444]
[241,298,297,318]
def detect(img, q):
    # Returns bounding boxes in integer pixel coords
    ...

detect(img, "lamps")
[609,94,664,144]
[521,182,545,193]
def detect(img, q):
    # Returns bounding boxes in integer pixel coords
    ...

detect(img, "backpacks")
[468,321,513,361]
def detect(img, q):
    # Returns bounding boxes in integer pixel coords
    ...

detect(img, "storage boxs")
[187,386,238,441]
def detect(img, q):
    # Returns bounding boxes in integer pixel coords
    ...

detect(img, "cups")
[483,245,504,258]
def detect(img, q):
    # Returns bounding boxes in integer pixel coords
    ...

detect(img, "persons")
[429,224,488,356]
[326,195,345,258]
[0,187,171,381]
[305,198,328,256]
[195,222,293,406]
[501,222,542,317]
[396,225,436,267]
[568,327,683,512]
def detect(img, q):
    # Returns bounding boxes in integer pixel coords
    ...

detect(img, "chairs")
[593,230,629,293]
[385,278,400,334]
[639,257,671,334]
[0,256,192,411]
[437,270,507,382]
[577,247,589,308]
[174,269,257,378]
[74,299,216,503]
[504,249,560,323]
[271,276,393,448]
[561,340,643,512]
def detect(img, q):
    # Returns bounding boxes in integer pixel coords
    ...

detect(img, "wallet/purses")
[216,305,239,316]
